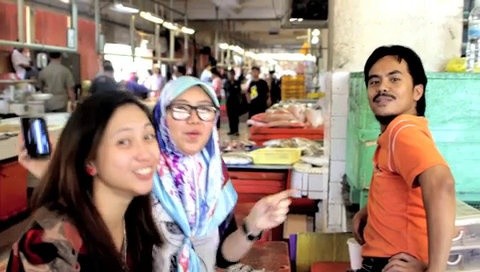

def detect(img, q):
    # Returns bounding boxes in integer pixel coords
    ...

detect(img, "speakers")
[290,0,328,21]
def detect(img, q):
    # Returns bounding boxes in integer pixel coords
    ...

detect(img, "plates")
[33,94,53,100]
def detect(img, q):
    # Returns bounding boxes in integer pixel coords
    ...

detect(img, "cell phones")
[21,117,51,158]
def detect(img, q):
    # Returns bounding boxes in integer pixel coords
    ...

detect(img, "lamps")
[109,0,245,55]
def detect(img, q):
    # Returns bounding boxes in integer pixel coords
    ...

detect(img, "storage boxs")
[446,199,480,272]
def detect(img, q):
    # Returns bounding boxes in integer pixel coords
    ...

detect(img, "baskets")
[250,148,302,164]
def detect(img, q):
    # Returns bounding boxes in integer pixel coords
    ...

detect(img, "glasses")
[168,103,217,121]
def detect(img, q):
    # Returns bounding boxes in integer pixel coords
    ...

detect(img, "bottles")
[466,0,480,72]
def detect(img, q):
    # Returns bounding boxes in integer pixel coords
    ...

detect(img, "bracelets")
[241,215,263,242]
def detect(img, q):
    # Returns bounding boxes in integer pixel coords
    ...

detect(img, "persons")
[36,51,77,113]
[200,58,282,137]
[88,60,187,100]
[11,39,39,81]
[5,92,164,272]
[17,76,298,272]
[346,45,456,272]
